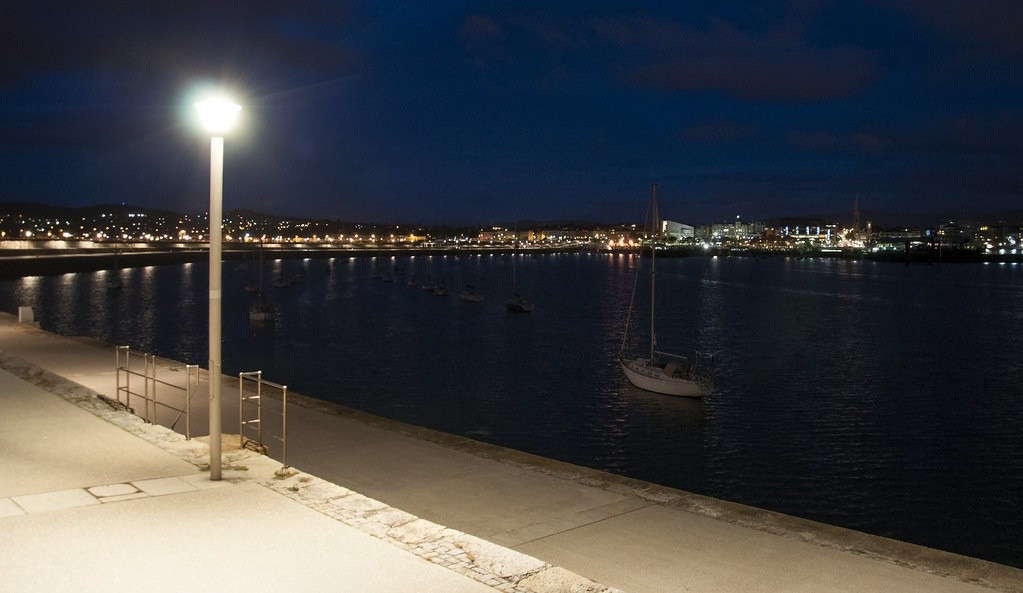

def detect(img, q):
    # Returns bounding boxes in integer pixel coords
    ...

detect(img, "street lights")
[278,221,308,251]
[196,95,241,481]
[939,230,944,257]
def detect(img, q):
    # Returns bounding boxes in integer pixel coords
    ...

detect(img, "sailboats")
[618,183,714,396]
